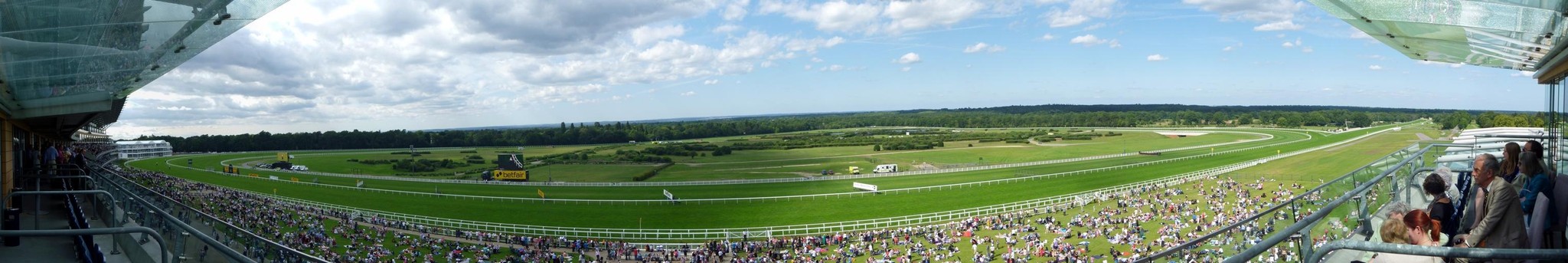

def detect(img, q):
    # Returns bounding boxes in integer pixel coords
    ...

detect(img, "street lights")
[410,145,415,177]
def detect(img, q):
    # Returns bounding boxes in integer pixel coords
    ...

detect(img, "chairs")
[61,175,107,262]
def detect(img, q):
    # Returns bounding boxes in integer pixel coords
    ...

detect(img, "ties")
[1483,190,1489,219]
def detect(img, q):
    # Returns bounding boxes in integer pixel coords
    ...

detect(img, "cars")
[255,155,308,171]
[176,151,247,154]
[650,141,678,144]
[1327,128,1348,132]
[822,170,834,175]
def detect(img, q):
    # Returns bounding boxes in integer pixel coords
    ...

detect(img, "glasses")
[1522,147,1531,152]
[1518,161,1523,166]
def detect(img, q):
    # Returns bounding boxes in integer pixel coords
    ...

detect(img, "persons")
[1361,201,1449,263]
[1510,140,1550,194]
[1422,173,1455,233]
[1497,142,1521,183]
[1434,166,1460,205]
[1516,151,1559,233]
[1403,209,1442,247]
[25,142,1384,263]
[1453,153,1530,263]
[1372,217,1445,263]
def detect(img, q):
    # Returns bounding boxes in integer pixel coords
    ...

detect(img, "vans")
[518,147,524,150]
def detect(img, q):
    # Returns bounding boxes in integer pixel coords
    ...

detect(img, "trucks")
[873,164,898,173]
[481,170,529,181]
[849,166,859,174]
